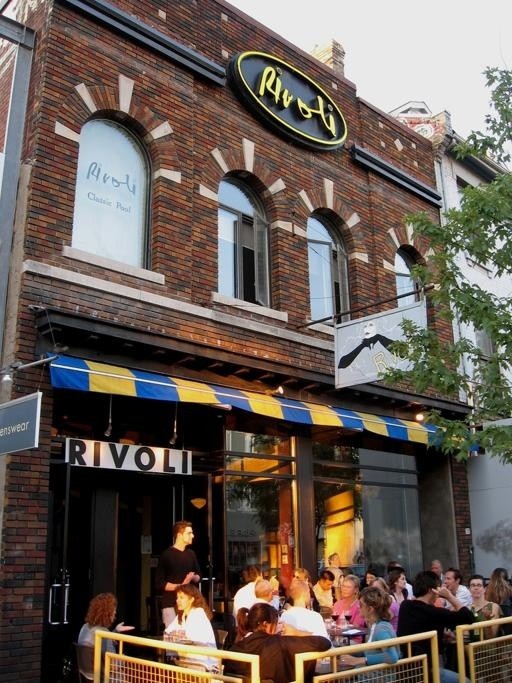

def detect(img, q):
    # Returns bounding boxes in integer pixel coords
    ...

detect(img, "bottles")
[470,605,481,640]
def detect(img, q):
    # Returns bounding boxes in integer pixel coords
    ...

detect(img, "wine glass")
[330,611,339,626]
[344,610,352,626]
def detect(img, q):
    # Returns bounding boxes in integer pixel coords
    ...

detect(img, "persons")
[78,591,135,683]
[237,553,512,682]
[157,521,204,627]
[164,584,220,674]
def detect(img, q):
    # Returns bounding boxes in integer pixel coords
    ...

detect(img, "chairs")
[73,643,104,683]
[319,606,332,619]
[211,612,235,650]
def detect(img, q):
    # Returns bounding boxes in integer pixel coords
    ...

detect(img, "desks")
[328,626,370,646]
[121,627,179,663]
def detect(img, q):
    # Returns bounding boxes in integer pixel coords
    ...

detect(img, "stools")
[146,596,163,635]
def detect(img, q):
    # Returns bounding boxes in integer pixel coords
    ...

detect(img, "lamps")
[170,402,178,444]
[104,394,112,436]
[266,387,283,396]
